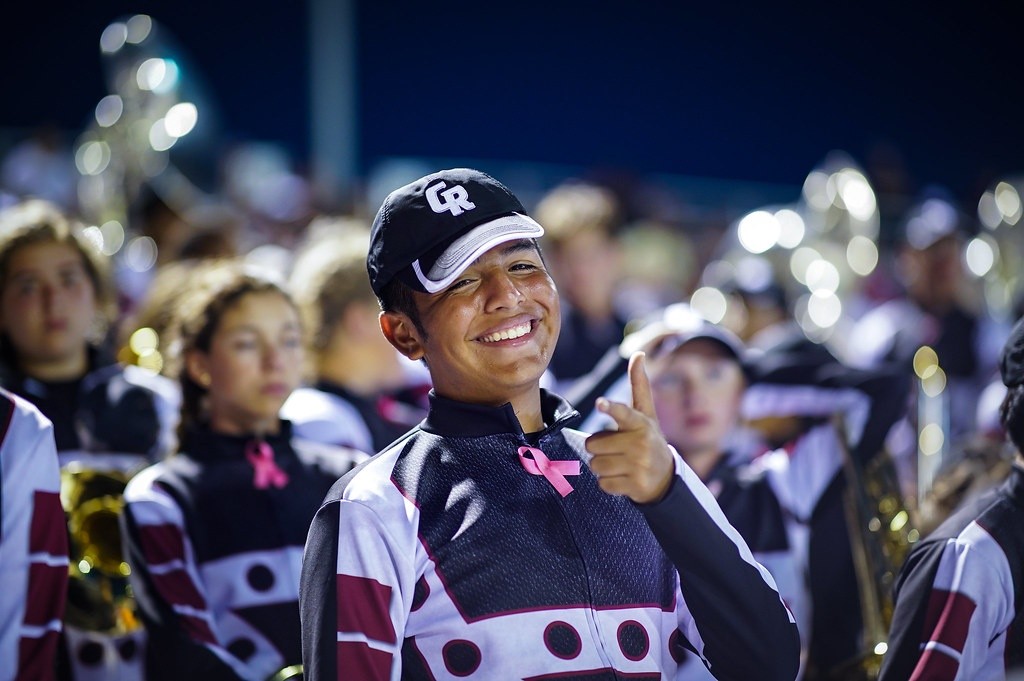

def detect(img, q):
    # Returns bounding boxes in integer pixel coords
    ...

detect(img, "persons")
[539,183,1024,681]
[1,201,430,681]
[878,317,1024,681]
[298,168,801,681]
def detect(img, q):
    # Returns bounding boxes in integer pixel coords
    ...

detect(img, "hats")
[1000,320,1024,391]
[644,314,745,382]
[366,167,545,300]
